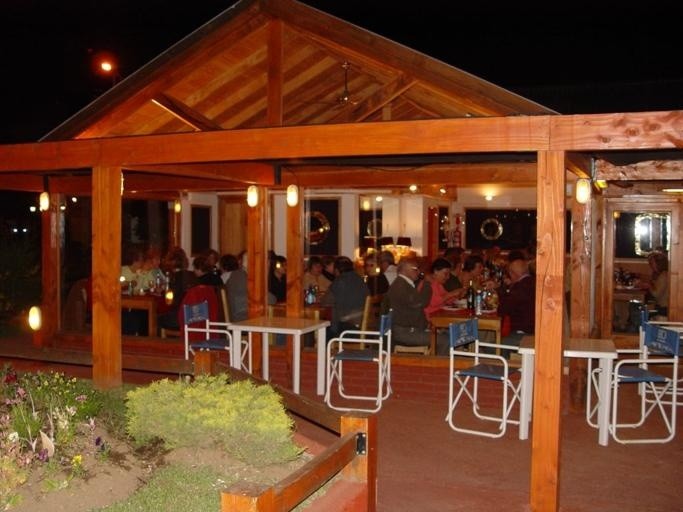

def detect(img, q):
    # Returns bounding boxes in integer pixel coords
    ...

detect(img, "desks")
[225,315,331,396]
[516,335,618,448]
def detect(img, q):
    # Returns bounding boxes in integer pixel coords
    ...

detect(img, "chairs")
[322,307,393,414]
[444,316,530,439]
[182,300,253,376]
[585,320,680,445]
[637,321,682,406]
[120,272,648,358]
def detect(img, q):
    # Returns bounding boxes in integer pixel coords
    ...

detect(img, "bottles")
[303,283,320,304]
[126,269,169,296]
[466,279,499,316]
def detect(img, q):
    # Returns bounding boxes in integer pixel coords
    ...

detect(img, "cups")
[615,277,640,290]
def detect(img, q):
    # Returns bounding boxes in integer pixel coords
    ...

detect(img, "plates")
[451,299,467,306]
[441,305,465,311]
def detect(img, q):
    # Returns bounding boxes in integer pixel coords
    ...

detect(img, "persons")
[121,247,537,358]
[636,252,669,321]
